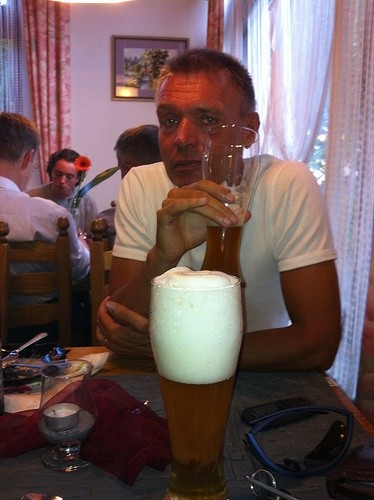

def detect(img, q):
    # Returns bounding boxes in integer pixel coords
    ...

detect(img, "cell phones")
[241,396,319,431]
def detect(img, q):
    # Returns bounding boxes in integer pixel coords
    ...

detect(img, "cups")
[199,123,260,289]
[149,272,243,500]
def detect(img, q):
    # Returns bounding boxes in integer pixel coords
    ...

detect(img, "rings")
[102,339,108,345]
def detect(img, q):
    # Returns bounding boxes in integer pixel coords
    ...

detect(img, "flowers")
[71,155,119,213]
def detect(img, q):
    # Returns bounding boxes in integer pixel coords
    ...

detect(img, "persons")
[114,124,163,180]
[0,111,92,343]
[96,49,341,374]
[26,149,99,235]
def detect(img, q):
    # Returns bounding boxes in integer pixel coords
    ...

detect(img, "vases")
[66,197,81,230]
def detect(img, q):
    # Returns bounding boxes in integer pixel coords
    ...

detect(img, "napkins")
[4,349,116,415]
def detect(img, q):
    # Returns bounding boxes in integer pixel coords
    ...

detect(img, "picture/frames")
[110,34,192,102]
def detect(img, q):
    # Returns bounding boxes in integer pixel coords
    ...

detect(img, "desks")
[0,347,374,500]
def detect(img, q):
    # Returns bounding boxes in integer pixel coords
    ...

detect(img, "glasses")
[245,469,300,500]
[247,404,354,478]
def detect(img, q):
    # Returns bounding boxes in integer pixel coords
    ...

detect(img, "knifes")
[2,332,48,367]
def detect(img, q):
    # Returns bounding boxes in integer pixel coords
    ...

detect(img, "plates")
[2,345,66,386]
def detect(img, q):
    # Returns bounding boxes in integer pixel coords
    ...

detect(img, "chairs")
[0,216,72,350]
[89,217,114,346]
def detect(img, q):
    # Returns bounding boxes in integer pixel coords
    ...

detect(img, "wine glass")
[36,358,99,473]
